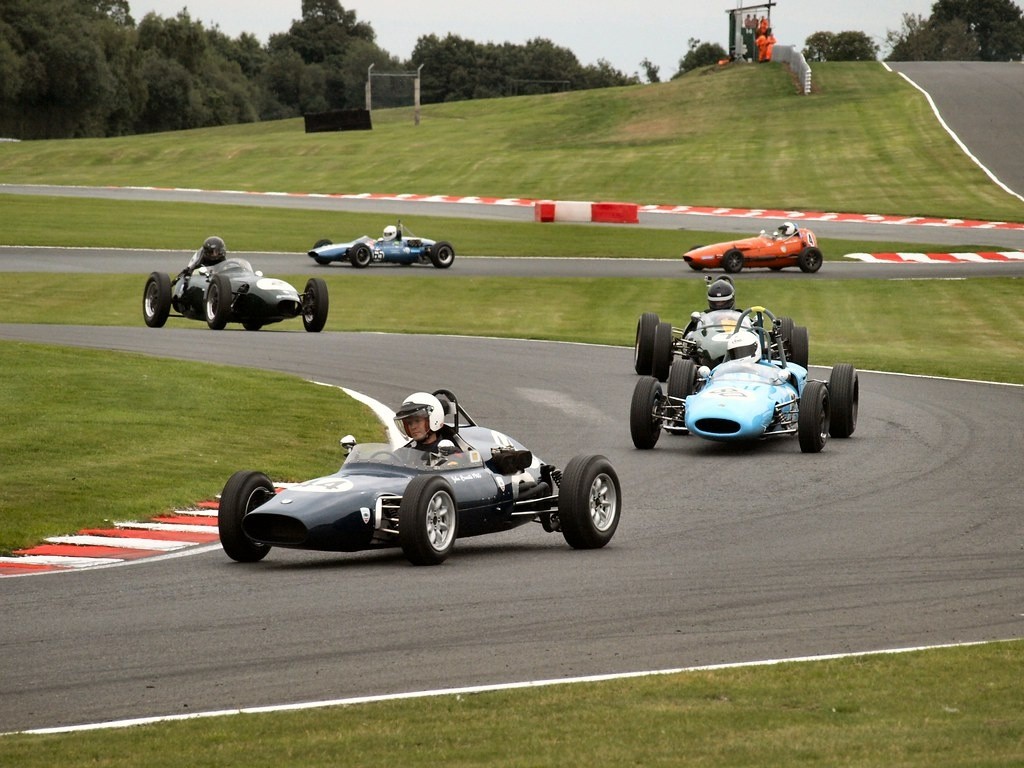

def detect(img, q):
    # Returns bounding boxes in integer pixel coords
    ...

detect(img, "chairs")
[435,424,469,454]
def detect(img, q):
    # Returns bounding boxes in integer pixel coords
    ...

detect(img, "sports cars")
[632,272,859,457]
[214,387,623,571]
[306,220,457,268]
[682,220,824,275]
[142,234,333,335]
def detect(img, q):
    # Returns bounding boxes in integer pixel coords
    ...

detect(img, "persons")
[760,16,767,34]
[745,14,751,34]
[708,280,735,311]
[752,14,759,32]
[383,225,397,241]
[755,33,777,63]
[393,391,461,464]
[195,236,226,268]
[778,222,795,240]
[727,330,763,364]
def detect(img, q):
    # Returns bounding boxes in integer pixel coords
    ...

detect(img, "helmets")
[382,225,397,241]
[201,237,226,265]
[707,279,736,310]
[778,222,795,236]
[394,392,445,440]
[726,331,762,363]
